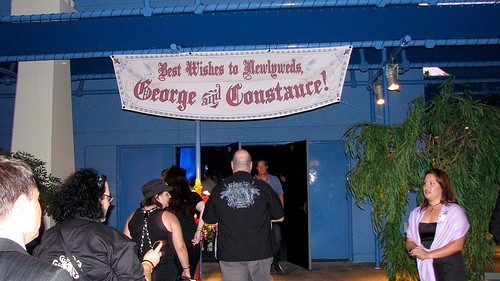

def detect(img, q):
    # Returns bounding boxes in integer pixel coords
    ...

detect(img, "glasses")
[102,192,116,203]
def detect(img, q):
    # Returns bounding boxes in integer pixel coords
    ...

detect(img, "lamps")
[386,63,400,90]
[373,76,385,105]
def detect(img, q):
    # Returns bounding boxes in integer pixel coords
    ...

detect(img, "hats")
[141,178,171,201]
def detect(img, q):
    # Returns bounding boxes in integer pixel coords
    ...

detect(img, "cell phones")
[152,240,164,251]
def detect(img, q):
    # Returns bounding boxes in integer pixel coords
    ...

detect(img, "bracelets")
[197,228,202,231]
[141,260,154,269]
[182,267,190,269]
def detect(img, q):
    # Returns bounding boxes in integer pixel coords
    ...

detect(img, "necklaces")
[428,202,441,214]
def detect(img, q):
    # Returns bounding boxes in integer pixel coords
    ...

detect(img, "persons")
[202,149,284,281]
[0,154,72,281]
[202,171,217,252]
[162,166,205,281]
[488,189,500,248]
[406,169,470,281]
[32,168,163,281]
[254,160,284,272]
[123,179,191,281]
